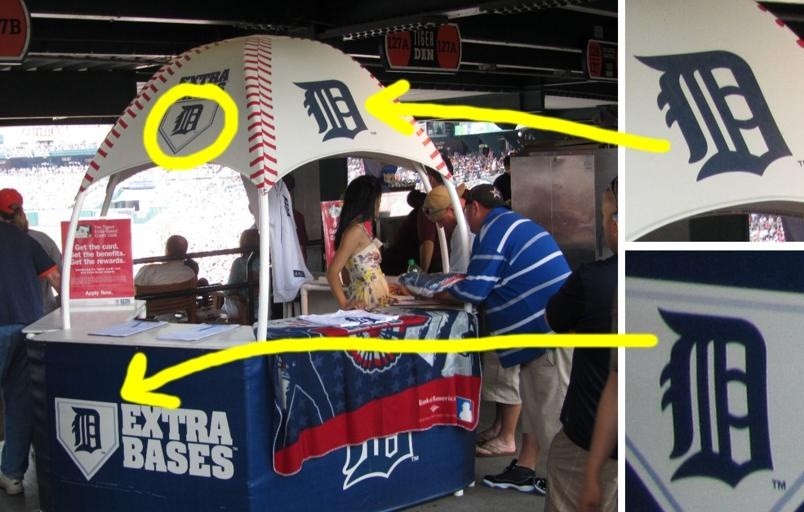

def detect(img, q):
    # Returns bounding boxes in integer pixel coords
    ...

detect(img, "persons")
[326,175,390,309]
[434,185,574,493]
[535,177,616,512]
[0,187,61,496]
[134,174,308,323]
[576,293,616,511]
[423,184,523,457]
[418,158,468,273]
[494,156,510,208]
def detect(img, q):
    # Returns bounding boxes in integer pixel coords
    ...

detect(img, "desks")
[268,306,474,512]
[21,300,146,332]
[25,324,255,512]
[300,279,472,315]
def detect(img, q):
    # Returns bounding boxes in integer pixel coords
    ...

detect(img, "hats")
[422,182,465,222]
[467,184,510,210]
[1,188,22,216]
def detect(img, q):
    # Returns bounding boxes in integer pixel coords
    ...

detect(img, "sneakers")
[482,458,534,495]
[0,469,25,496]
[532,478,547,495]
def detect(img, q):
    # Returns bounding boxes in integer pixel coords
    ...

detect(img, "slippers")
[476,432,518,456]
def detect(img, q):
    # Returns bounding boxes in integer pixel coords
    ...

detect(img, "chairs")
[135,277,198,323]
[207,269,259,323]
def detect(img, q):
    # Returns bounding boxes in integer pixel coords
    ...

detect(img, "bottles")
[407,260,422,274]
